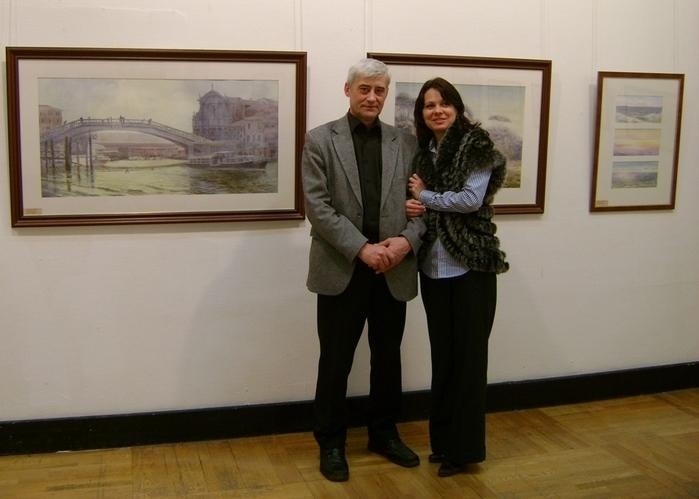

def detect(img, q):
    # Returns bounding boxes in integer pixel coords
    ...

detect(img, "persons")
[406,77,511,478]
[300,58,431,482]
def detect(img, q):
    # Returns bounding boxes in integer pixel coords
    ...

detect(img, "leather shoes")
[366,429,419,468]
[318,440,350,482]
[427,451,466,477]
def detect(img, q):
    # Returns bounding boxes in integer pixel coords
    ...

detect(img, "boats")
[183,148,268,169]
